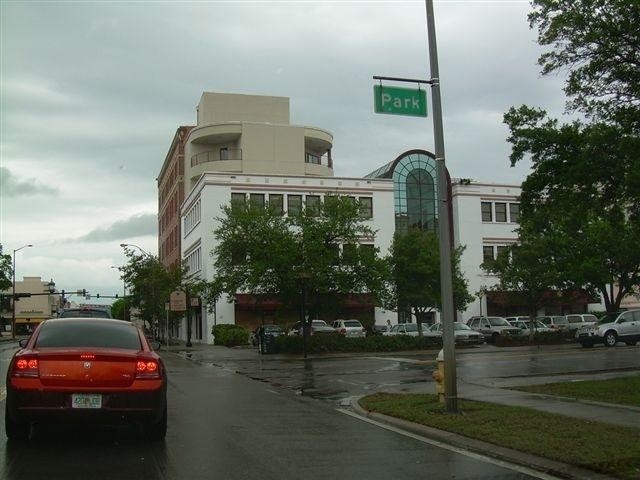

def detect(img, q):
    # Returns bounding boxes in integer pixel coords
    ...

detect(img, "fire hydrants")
[432,348,444,403]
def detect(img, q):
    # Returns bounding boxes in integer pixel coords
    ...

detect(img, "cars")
[535,316,570,336]
[6,318,169,441]
[507,315,554,336]
[428,322,485,345]
[564,314,599,332]
[250,325,286,346]
[332,320,367,339]
[382,323,439,339]
[288,319,339,336]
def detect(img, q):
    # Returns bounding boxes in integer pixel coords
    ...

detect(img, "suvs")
[575,309,640,348]
[465,317,523,338]
[53,306,113,321]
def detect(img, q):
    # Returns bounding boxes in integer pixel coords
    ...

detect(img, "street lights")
[120,243,157,342]
[111,262,127,320]
[11,243,37,338]
